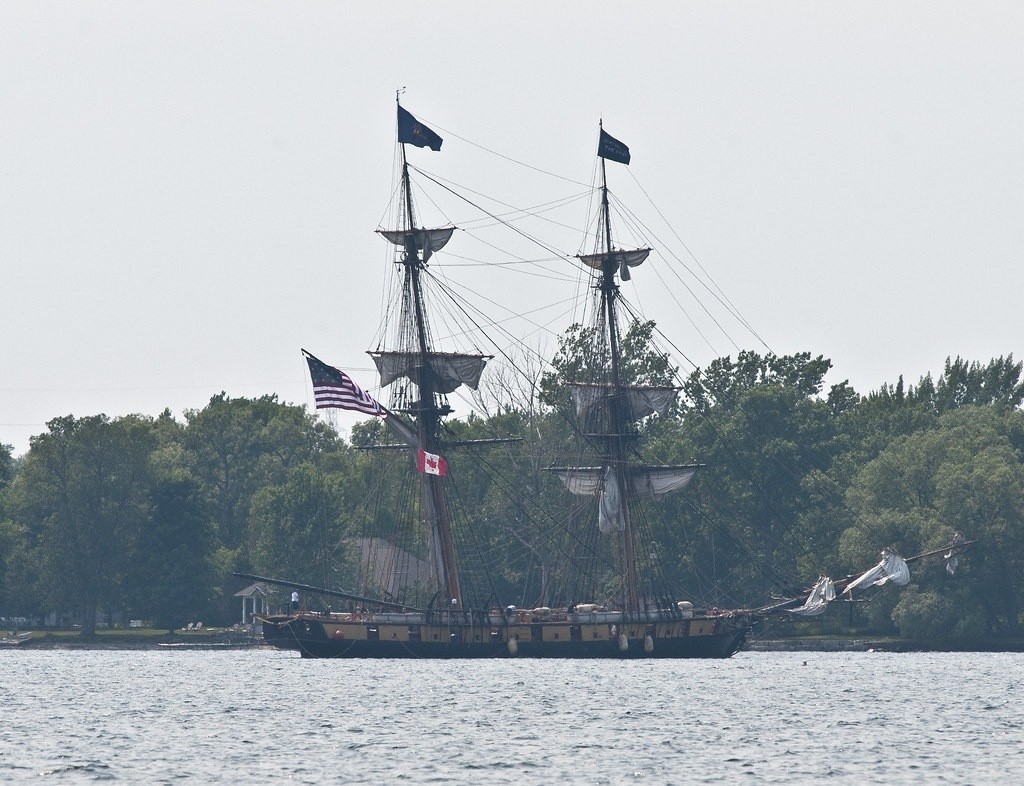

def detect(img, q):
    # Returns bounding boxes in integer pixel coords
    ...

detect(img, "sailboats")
[231,82,980,659]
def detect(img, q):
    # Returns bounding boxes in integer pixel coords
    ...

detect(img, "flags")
[418,449,447,476]
[306,357,386,416]
[397,106,443,151]
[597,130,630,165]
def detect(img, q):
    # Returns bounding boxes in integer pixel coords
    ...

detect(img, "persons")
[506,605,516,617]
[291,590,299,612]
[567,600,575,614]
